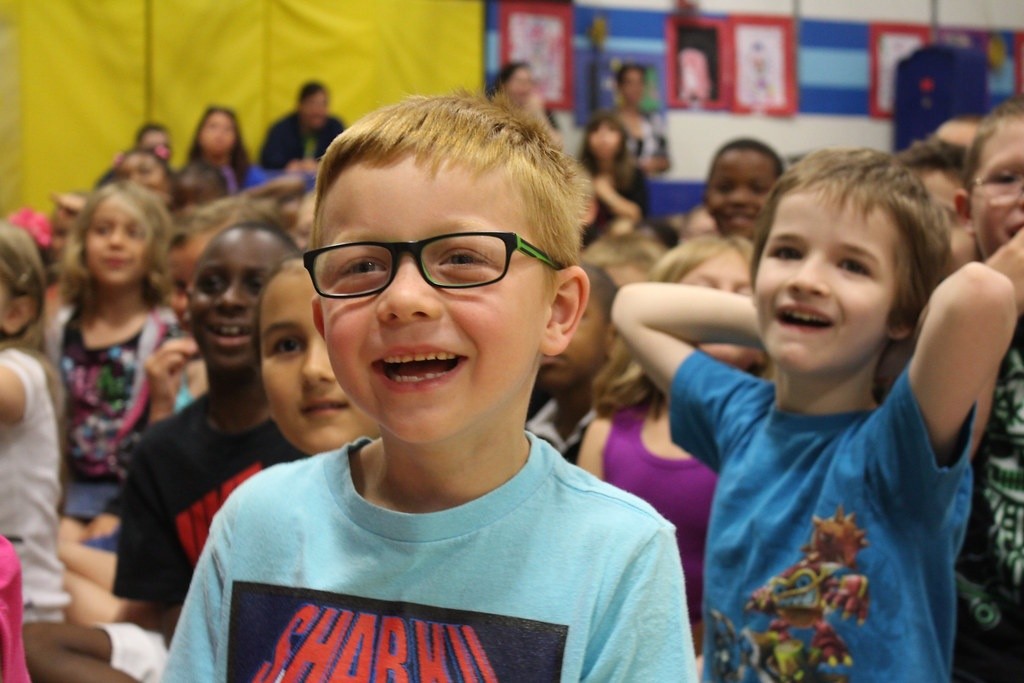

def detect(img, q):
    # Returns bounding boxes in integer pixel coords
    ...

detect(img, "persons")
[579,110,647,249]
[604,146,1018,683]
[156,96,699,683]
[17,223,300,683]
[702,139,784,238]
[142,187,299,424]
[259,80,345,174]
[251,254,380,453]
[576,234,767,658]
[0,223,70,624]
[42,180,182,554]
[95,66,1023,682]
[528,258,623,464]
[186,106,249,196]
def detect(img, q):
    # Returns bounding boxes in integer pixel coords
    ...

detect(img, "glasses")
[302,232,562,300]
[966,171,1024,197]
